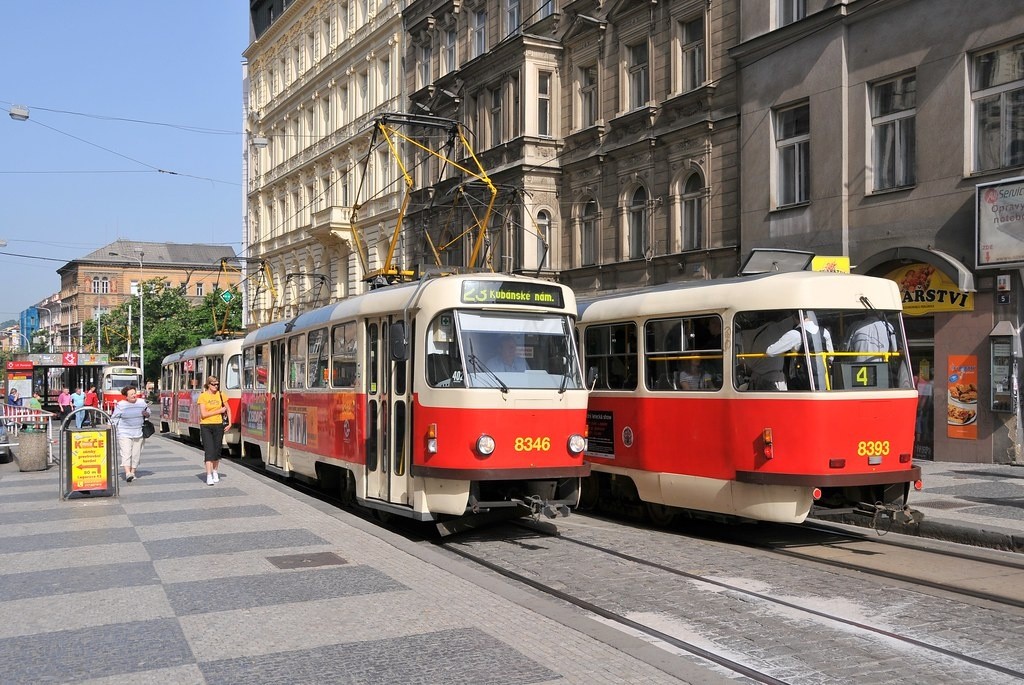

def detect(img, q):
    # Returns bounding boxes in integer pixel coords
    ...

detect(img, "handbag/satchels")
[141,421,155,438]
[221,408,229,426]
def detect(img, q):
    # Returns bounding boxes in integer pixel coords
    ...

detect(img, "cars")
[0,420,10,464]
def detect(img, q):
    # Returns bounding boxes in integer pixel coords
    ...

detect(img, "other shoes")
[207,475,214,486]
[125,473,138,482]
[212,471,219,482]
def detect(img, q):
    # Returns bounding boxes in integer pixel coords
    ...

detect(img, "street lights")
[73,284,101,354]
[52,302,71,352]
[29,306,52,353]
[109,252,145,400]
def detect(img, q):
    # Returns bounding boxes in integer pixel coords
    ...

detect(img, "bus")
[49,365,143,423]
[240,272,598,542]
[574,270,923,539]
[160,336,244,456]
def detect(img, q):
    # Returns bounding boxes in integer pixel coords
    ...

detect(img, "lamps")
[9,106,30,121]
[252,137,268,148]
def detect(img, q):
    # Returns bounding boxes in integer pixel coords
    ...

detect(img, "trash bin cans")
[18,421,47,472]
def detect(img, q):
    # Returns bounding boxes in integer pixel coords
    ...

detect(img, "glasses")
[210,383,219,386]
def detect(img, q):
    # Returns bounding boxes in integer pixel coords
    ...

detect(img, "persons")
[108,386,151,482]
[81,385,98,428]
[200,376,232,485]
[485,335,530,373]
[71,388,86,429]
[735,311,796,390]
[9,388,21,406]
[838,310,897,363]
[679,347,715,391]
[58,387,73,430]
[766,309,834,391]
[33,391,41,401]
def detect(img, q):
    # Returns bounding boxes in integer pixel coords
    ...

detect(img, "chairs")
[428,354,460,385]
[478,334,499,363]
[673,371,681,390]
